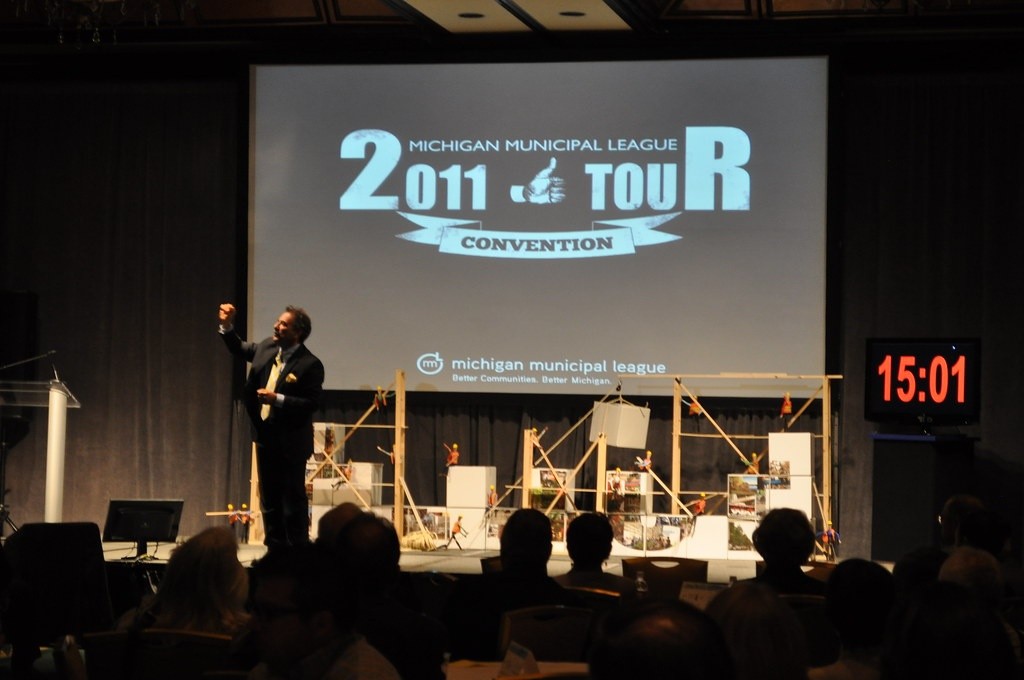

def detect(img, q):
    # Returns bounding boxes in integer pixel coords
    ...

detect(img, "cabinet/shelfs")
[505,375,842,563]
[248,368,405,546]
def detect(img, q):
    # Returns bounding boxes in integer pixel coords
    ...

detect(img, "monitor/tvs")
[102,499,184,543]
[865,336,981,425]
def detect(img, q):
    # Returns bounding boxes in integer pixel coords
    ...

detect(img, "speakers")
[0,522,113,646]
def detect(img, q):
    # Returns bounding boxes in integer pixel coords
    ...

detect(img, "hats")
[310,503,374,547]
[754,509,814,566]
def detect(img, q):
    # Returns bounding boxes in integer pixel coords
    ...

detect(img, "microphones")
[0,350,59,381]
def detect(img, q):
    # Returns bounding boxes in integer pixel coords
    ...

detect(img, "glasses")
[244,595,338,625]
[936,514,957,526]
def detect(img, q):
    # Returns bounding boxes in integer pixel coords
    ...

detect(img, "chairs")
[0,524,830,680]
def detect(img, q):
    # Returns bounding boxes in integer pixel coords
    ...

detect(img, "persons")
[218,304,326,568]
[0,495,1024,680]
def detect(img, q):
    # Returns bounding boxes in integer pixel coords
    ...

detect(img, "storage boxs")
[304,401,815,560]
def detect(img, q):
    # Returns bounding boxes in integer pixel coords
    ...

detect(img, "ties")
[260,352,283,421]
